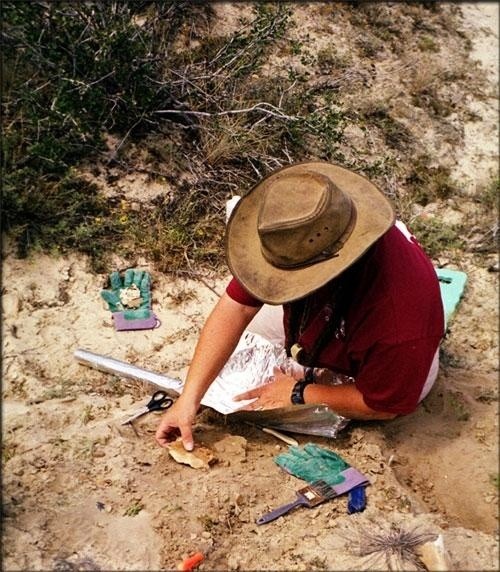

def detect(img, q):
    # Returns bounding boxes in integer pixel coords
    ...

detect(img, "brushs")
[257,480,337,525]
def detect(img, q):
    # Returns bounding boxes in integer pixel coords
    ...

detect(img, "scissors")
[121,390,173,426]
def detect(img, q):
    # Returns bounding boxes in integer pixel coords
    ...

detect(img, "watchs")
[289,379,312,406]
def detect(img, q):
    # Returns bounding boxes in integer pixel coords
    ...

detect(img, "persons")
[152,161,450,461]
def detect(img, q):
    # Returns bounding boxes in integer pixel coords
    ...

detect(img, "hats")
[224,160,397,306]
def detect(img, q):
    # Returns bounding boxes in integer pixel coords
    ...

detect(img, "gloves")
[272,443,371,501]
[99,268,161,332]
